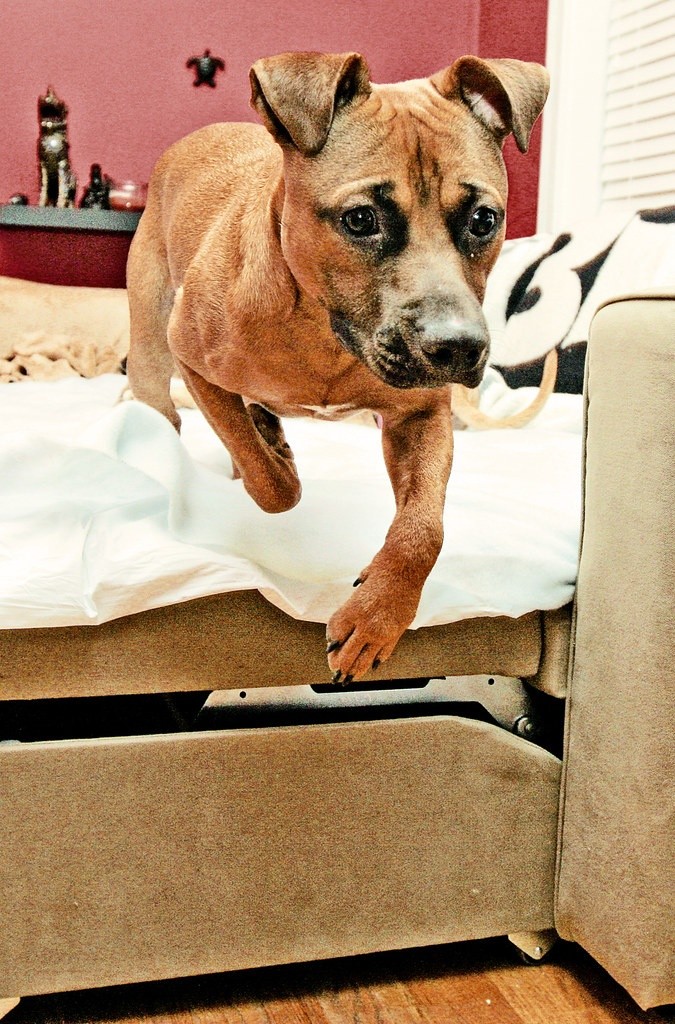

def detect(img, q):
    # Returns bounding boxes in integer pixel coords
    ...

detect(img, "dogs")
[32,82,77,209]
[127,50,551,690]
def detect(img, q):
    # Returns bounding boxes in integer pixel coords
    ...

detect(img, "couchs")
[1,217,675,1024]
